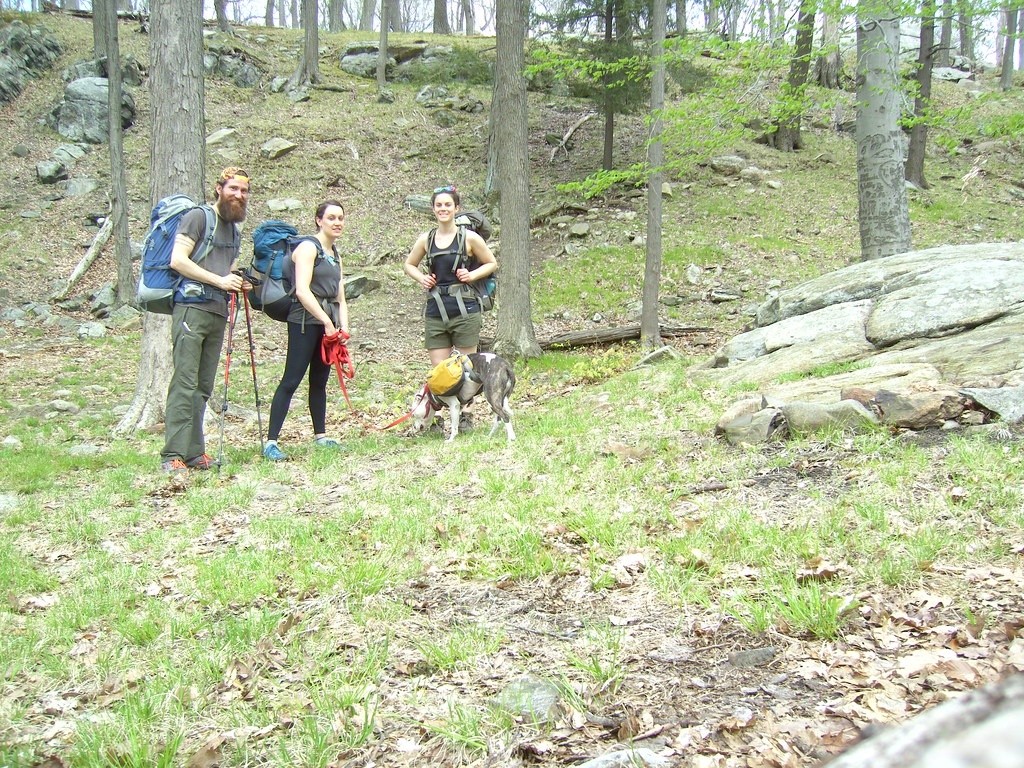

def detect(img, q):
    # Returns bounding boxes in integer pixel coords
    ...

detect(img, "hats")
[220,166,249,183]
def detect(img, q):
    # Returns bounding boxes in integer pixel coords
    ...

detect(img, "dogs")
[409,353,517,441]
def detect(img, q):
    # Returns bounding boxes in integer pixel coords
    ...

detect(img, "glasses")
[434,185,455,193]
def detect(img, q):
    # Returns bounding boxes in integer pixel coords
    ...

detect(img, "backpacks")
[136,194,242,314]
[245,219,339,321]
[428,210,496,313]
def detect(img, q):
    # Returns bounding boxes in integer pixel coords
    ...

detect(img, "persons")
[404,190,497,433]
[261,199,351,460]
[160,167,253,478]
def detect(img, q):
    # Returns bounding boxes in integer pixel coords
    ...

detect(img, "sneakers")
[314,436,337,447]
[263,442,287,462]
[456,412,475,432]
[430,415,444,432]
[185,453,220,471]
[161,459,188,478]
